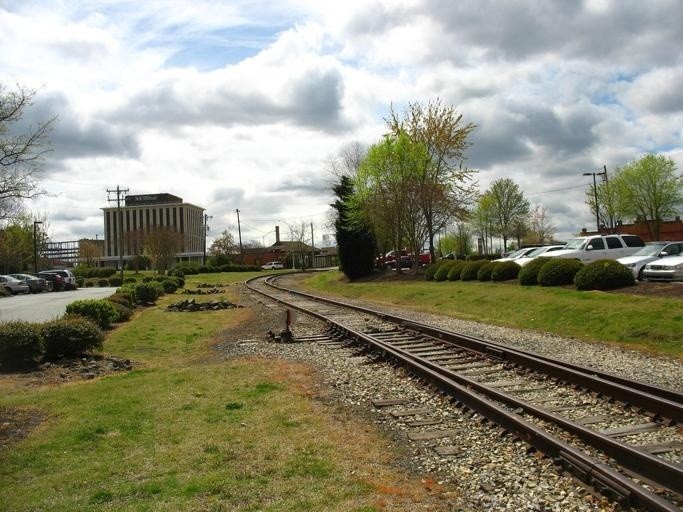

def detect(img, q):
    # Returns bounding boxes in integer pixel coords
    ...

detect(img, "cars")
[492,245,565,268]
[614,241,683,282]
[385,256,423,270]
[0,273,53,296]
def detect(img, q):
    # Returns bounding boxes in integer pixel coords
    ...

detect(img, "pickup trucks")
[376,250,430,264]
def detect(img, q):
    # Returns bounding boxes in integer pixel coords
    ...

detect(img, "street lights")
[34,221,42,273]
[583,165,613,233]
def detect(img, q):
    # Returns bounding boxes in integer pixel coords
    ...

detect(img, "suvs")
[539,234,645,265]
[261,262,284,271]
[32,270,75,291]
